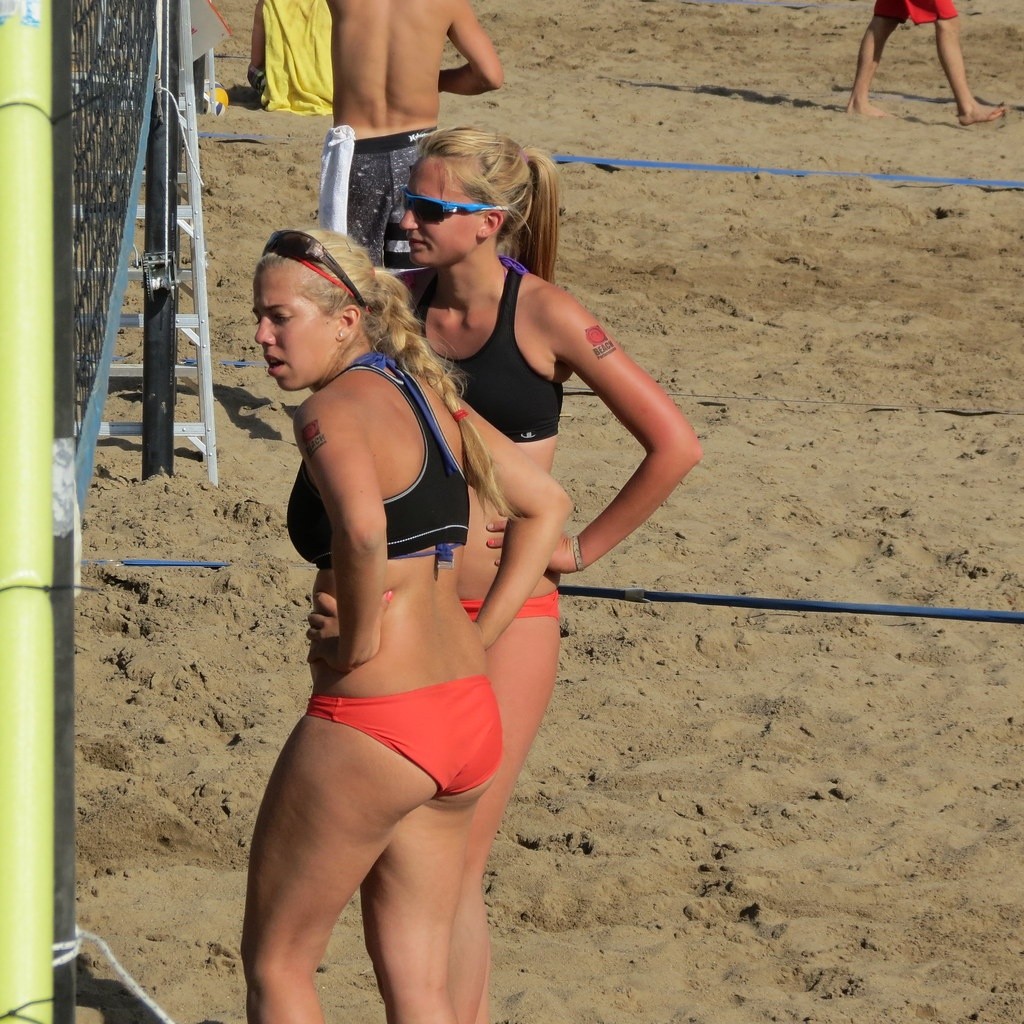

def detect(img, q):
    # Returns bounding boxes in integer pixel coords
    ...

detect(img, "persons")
[326,0,504,278]
[241,230,575,1024]
[391,127,702,1024]
[847,0,1005,126]
[247,0,267,103]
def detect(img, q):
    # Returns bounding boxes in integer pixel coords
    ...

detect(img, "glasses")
[402,185,510,221]
[263,229,368,309]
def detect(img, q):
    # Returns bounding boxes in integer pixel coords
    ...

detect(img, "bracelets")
[573,536,584,570]
